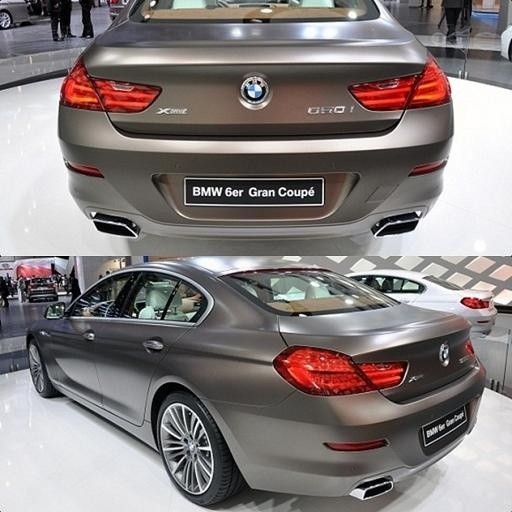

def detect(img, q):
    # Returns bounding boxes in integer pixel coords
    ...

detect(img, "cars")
[339,265,500,339]
[53,0,458,242]
[0,0,37,30]
[25,277,59,302]
[498,23,512,60]
[23,255,490,511]
[109,0,131,21]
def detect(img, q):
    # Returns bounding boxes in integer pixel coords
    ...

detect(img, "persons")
[79,1,94,39]
[96,274,107,301]
[47,0,59,41]
[441,0,464,40]
[0,274,81,306]
[105,271,113,300]
[59,0,77,41]
[460,0,475,34]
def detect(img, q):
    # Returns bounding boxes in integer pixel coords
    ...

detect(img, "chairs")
[305,280,325,299]
[171,0,207,9]
[300,0,335,8]
[370,279,393,291]
[143,283,187,321]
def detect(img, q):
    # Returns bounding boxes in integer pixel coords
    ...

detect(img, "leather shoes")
[53,33,91,41]
